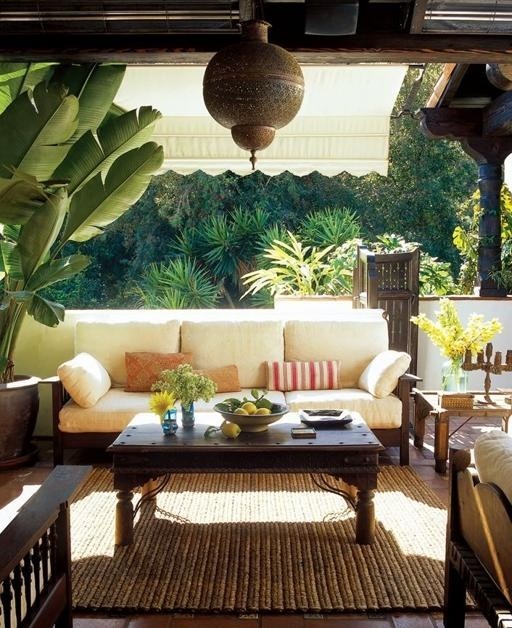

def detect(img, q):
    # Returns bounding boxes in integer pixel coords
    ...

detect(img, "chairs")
[443,445,512,627]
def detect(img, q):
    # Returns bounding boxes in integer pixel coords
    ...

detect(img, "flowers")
[145,391,179,429]
[151,358,218,410]
[409,294,503,387]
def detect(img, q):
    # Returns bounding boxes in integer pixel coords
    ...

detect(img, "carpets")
[67,459,483,613]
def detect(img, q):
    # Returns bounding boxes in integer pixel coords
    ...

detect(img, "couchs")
[36,314,425,466]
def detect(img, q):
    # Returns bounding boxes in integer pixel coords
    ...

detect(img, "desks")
[411,386,512,472]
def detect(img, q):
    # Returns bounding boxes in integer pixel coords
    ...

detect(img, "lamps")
[201,17,307,172]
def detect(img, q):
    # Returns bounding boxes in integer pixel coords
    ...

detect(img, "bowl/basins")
[213,400,289,433]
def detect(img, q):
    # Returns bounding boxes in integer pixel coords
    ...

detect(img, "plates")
[300,408,352,430]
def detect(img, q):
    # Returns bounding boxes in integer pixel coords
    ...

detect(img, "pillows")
[192,362,243,393]
[356,348,413,398]
[474,428,512,506]
[122,350,192,393]
[264,360,342,391]
[55,351,112,408]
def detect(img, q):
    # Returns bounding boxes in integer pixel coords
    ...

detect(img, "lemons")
[221,422,242,439]
[253,408,271,416]
[241,401,257,413]
[234,407,249,415]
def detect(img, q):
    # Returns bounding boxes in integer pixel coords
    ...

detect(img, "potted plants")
[0,58,167,468]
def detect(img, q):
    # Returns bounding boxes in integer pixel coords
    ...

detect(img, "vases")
[441,358,468,392]
[161,406,178,436]
[180,400,194,428]
